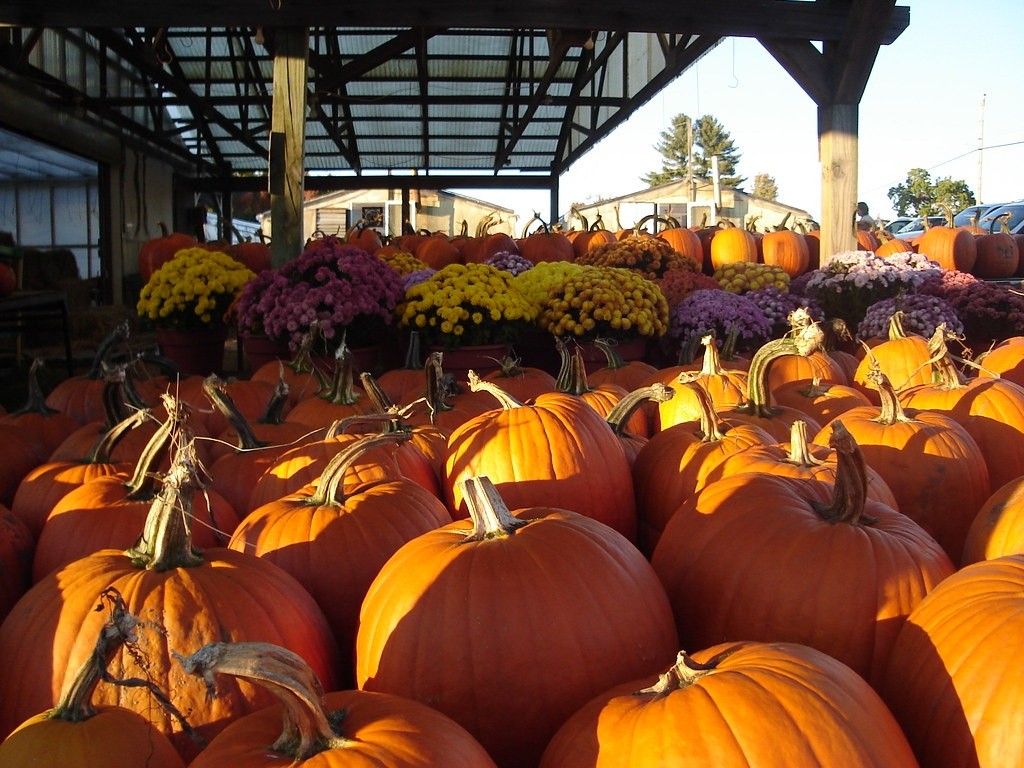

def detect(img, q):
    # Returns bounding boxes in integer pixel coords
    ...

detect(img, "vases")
[375,333,399,369]
[397,335,411,365]
[514,334,561,376]
[157,328,228,376]
[290,341,386,386]
[237,332,290,370]
[426,342,514,389]
[565,340,646,371]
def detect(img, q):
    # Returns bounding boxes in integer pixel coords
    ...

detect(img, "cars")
[942,203,1005,229]
[880,216,914,240]
[890,217,950,241]
[972,202,1024,235]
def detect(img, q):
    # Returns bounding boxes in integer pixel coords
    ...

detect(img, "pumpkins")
[0,307,1024,768]
[0,260,16,299]
[140,206,1024,293]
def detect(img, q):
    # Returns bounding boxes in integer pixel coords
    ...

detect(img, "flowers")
[221,267,280,339]
[713,262,792,294]
[746,284,826,328]
[669,287,774,353]
[941,271,979,296]
[887,251,941,292]
[538,265,671,343]
[402,269,436,289]
[803,248,896,320]
[919,276,940,294]
[958,278,1024,333]
[394,262,539,351]
[380,251,431,276]
[516,258,574,302]
[793,269,819,295]
[131,247,258,329]
[654,268,723,306]
[853,293,965,346]
[270,245,406,350]
[578,232,704,278]
